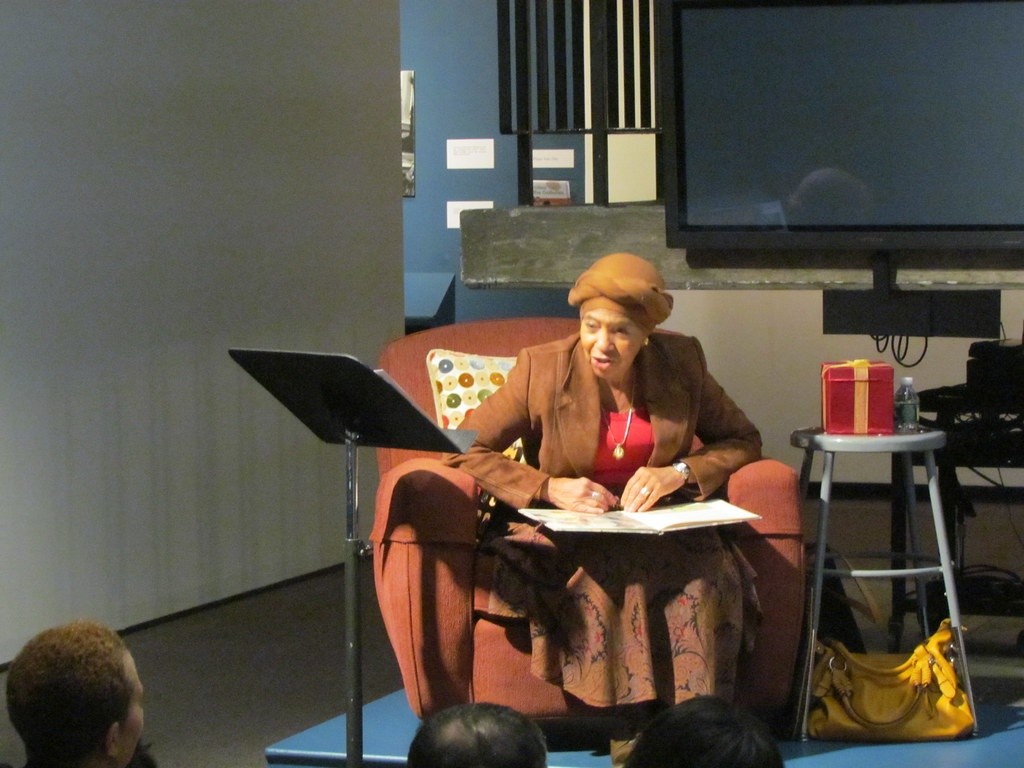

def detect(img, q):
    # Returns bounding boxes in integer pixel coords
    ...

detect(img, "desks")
[791,427,979,742]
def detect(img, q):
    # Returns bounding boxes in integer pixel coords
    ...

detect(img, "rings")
[641,487,649,496]
[592,492,600,499]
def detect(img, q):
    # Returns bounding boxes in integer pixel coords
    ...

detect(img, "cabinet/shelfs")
[889,383,1023,656]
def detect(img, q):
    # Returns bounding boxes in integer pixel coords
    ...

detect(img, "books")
[517,498,761,536]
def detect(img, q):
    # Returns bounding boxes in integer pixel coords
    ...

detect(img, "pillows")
[425,348,530,540]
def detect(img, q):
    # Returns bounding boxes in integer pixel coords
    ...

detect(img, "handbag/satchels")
[808,621,975,741]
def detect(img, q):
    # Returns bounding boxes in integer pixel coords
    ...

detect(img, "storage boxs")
[819,360,895,435]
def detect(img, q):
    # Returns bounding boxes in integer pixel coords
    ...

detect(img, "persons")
[406,702,548,768]
[445,256,763,768]
[625,694,784,768]
[5,619,158,768]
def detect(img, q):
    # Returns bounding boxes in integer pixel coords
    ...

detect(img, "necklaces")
[601,377,634,460]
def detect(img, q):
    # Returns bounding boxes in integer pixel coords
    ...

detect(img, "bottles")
[893,377,918,435]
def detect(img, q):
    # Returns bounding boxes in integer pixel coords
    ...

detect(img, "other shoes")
[608,704,655,768]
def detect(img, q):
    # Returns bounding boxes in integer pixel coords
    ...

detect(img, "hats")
[567,254,676,335]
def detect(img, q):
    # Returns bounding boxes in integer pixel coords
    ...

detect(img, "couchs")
[369,318,807,732]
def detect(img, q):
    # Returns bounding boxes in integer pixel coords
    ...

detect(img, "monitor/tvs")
[653,0,1024,252]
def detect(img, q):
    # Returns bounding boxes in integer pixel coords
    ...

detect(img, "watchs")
[672,462,690,485]
